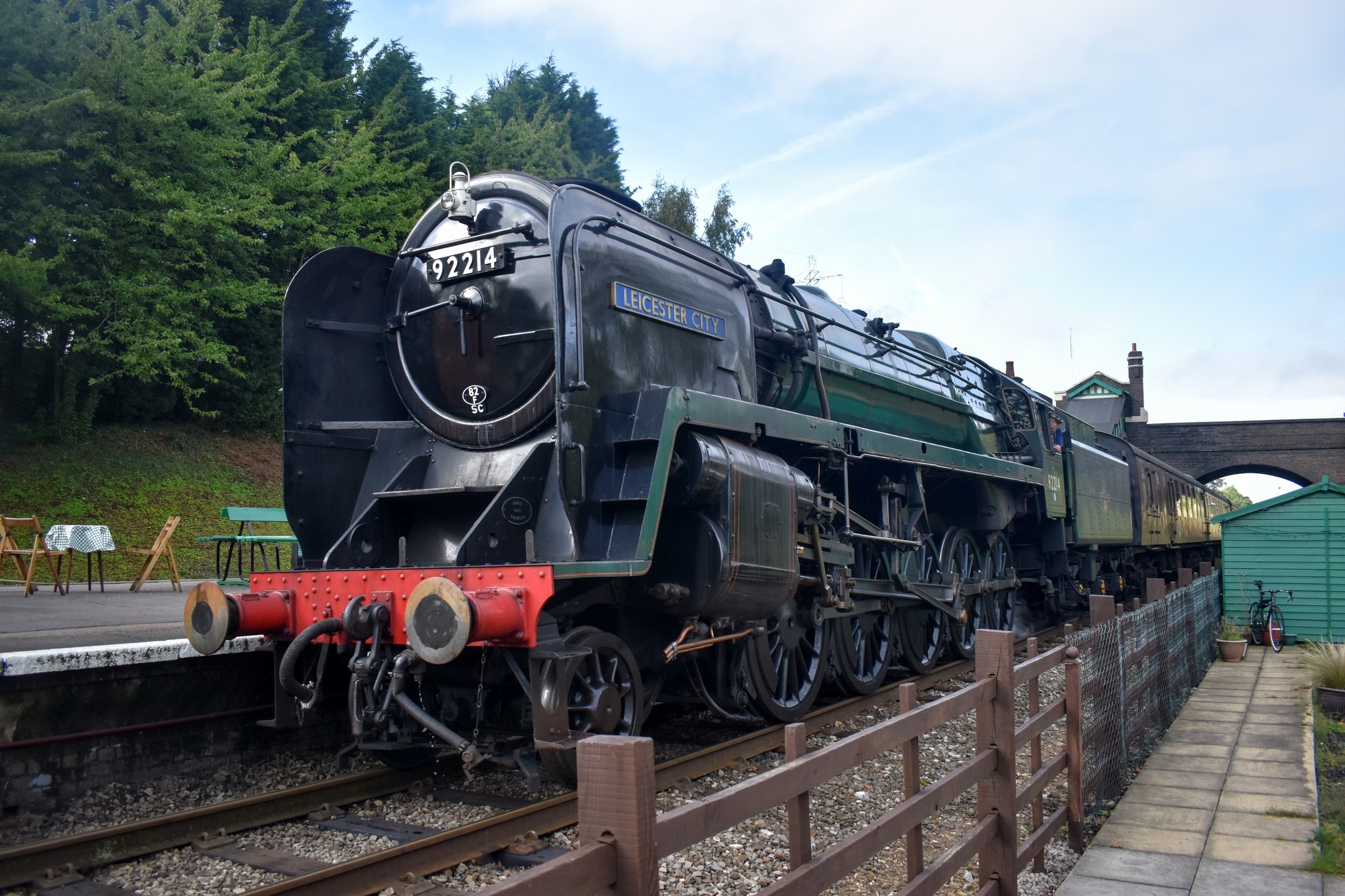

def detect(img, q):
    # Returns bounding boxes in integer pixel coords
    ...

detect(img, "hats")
[1050,414,1063,423]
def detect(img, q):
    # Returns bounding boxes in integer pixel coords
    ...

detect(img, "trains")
[183,159,1234,793]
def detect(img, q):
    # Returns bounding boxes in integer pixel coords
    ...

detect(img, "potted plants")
[1212,614,1247,662]
[1256,619,1281,646]
[1230,626,1252,645]
[1287,628,1345,719]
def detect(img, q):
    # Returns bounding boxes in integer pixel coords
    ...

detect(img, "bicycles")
[1248,580,1294,652]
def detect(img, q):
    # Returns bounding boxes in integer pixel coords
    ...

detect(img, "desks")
[45,525,115,594]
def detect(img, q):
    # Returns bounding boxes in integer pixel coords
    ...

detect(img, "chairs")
[127,516,183,593]
[0,515,66,597]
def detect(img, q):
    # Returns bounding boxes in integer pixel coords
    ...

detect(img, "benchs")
[196,507,302,586]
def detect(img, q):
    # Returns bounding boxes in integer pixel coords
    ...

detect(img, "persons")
[1049,414,1064,451]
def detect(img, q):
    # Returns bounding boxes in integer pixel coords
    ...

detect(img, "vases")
[1281,633,1297,646]
